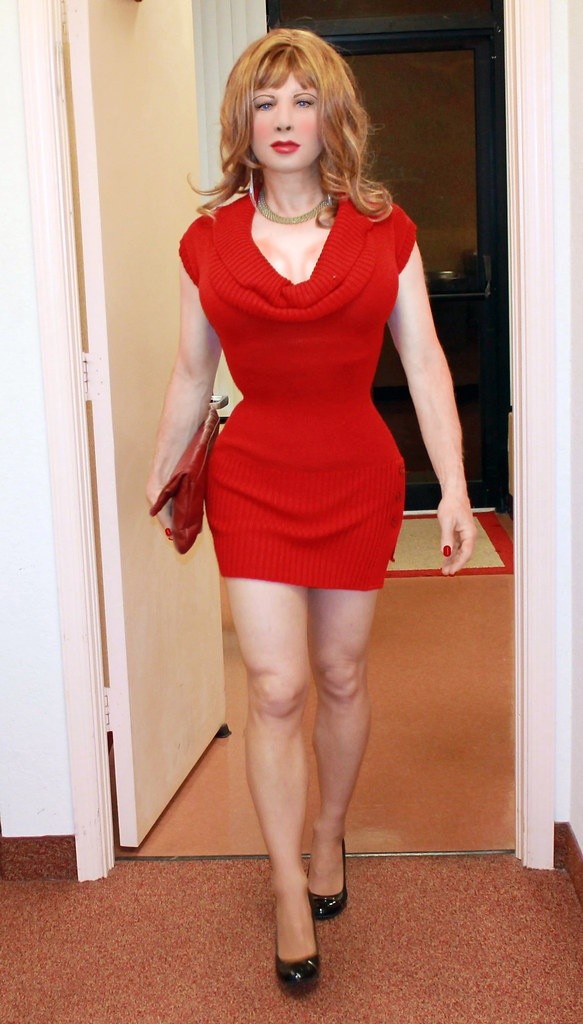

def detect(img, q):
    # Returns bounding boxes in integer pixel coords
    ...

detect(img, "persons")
[144,29,480,987]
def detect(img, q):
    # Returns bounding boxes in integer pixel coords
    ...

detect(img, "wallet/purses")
[148,409,221,555]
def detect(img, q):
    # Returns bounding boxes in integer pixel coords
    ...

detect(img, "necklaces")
[257,190,332,224]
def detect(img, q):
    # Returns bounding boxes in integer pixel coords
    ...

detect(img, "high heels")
[306,837,347,920]
[275,887,321,984]
